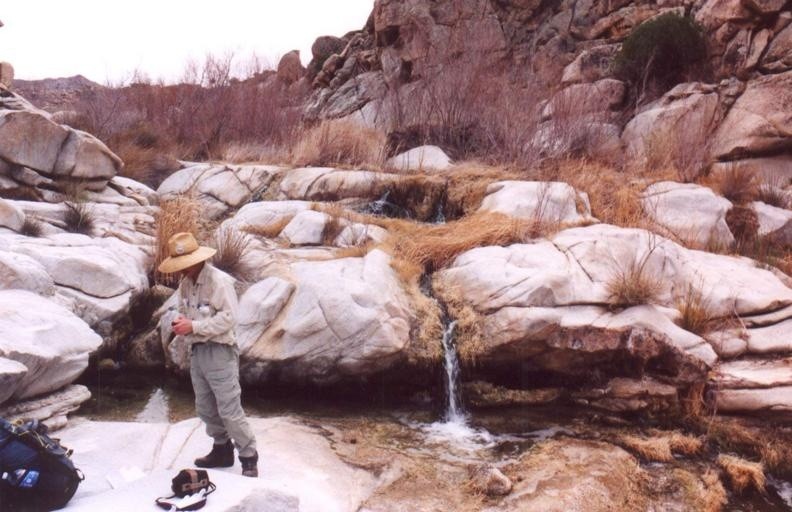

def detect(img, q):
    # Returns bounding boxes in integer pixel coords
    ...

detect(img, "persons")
[157,232,257,477]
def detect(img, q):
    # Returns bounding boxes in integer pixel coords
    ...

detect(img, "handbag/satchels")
[0,418,84,512]
[173,470,210,498]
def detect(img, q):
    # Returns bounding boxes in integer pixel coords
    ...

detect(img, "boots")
[239,451,258,477]
[195,439,234,468]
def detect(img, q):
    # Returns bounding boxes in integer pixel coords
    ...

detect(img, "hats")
[158,232,216,273]
